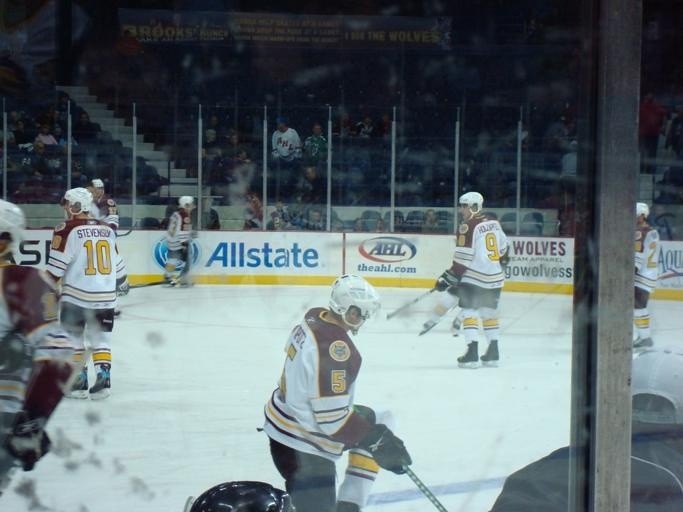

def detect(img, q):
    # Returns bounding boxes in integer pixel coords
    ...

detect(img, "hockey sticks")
[386,285,436,321]
[115,230,133,236]
[128,281,170,290]
[419,303,459,336]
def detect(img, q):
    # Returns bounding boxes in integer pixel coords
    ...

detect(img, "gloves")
[362,423,413,474]
[435,270,460,291]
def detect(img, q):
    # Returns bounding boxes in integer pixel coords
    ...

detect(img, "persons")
[45,186,130,396]
[631,202,660,350]
[430,189,511,364]
[424,286,468,333]
[1,83,683,238]
[265,273,416,512]
[162,195,197,287]
[488,346,683,512]
[0,198,78,496]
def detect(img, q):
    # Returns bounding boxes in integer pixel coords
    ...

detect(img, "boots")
[165,276,181,289]
[457,341,479,361]
[70,367,90,390]
[480,340,499,361]
[89,362,110,393]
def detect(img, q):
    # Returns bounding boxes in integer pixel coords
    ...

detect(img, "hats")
[631,345,683,425]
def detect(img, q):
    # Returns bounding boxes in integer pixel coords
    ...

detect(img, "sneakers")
[632,335,654,348]
[453,318,461,330]
[424,320,435,329]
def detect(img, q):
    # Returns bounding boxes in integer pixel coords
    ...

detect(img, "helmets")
[459,191,483,215]
[63,187,93,215]
[0,200,26,259]
[178,196,194,210]
[328,274,380,336]
[637,202,651,220]
[90,178,108,196]
